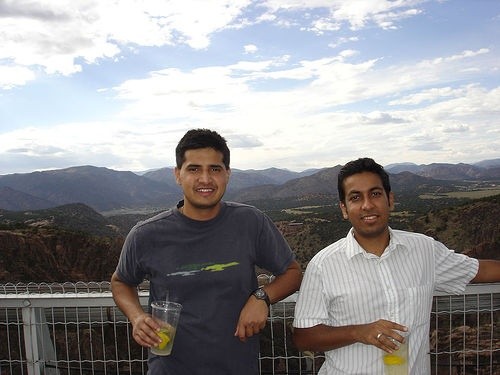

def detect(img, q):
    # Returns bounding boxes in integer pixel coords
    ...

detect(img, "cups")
[150,301,182,356]
[382,337,409,375]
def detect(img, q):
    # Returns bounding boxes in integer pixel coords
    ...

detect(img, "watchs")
[250,288,271,307]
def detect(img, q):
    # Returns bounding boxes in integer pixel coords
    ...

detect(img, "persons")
[294,158,500,375]
[110,129,303,375]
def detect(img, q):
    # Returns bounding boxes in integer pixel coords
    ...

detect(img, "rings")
[376,333,383,339]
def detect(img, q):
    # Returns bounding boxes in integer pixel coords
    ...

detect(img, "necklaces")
[180,205,184,213]
